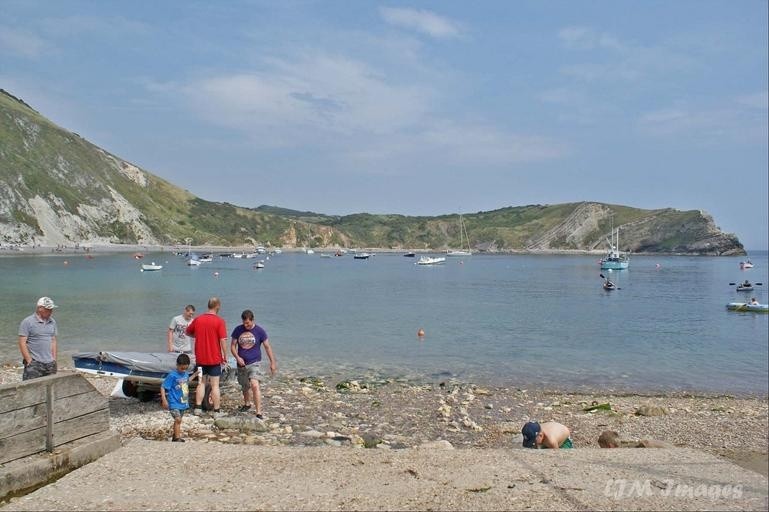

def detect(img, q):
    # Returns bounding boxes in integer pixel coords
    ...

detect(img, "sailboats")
[446,210,473,256]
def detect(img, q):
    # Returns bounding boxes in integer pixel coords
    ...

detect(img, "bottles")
[197,366,203,384]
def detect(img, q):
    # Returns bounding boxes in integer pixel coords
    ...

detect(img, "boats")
[741,263,754,268]
[416,255,447,265]
[600,215,631,270]
[726,302,769,313]
[171,247,284,269]
[736,284,754,292]
[354,253,370,260]
[71,350,239,410]
[601,278,616,291]
[142,263,163,271]
[403,254,416,257]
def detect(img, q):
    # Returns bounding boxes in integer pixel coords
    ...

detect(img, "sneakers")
[240,405,251,412]
[256,414,263,419]
[214,411,229,418]
[172,432,185,442]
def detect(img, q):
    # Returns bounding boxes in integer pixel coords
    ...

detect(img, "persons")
[18,297,58,381]
[519,420,574,448]
[160,354,199,442]
[604,278,611,285]
[167,305,195,353]
[31,242,90,253]
[741,280,750,287]
[747,257,751,265]
[230,310,277,418]
[186,298,228,414]
[751,298,758,305]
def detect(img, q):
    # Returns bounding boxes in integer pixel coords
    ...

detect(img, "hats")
[522,422,539,447]
[36,296,59,310]
